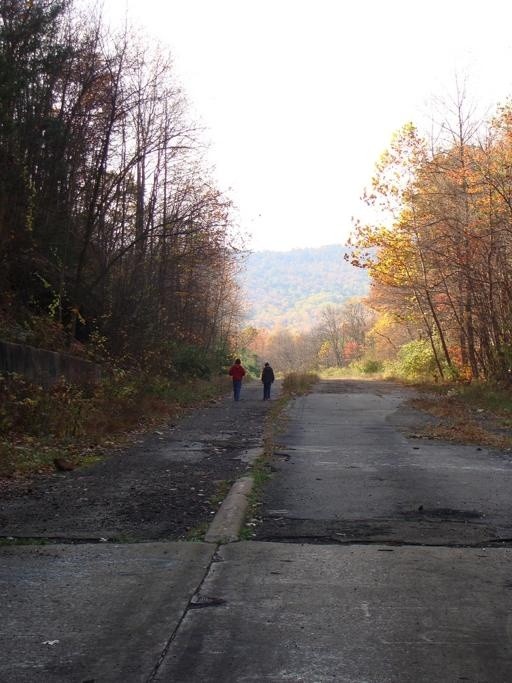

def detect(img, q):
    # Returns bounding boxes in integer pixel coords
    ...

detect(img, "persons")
[262,362,274,401]
[229,359,245,401]
